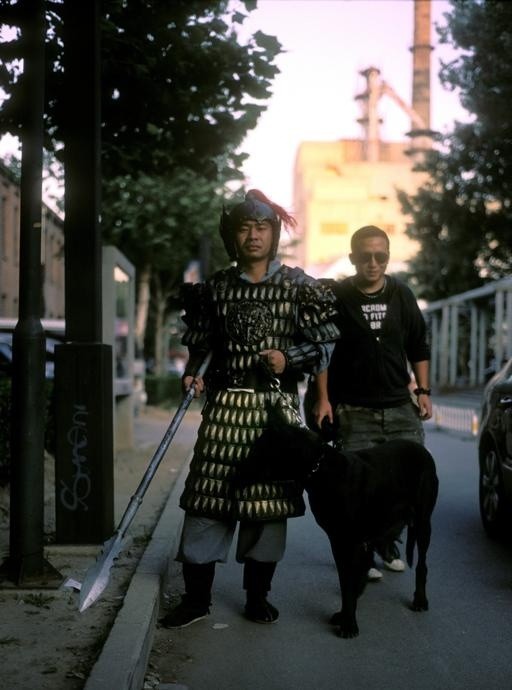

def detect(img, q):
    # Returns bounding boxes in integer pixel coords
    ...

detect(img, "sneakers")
[369,552,406,578]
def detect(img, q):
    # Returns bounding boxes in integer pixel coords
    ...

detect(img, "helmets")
[219,193,280,261]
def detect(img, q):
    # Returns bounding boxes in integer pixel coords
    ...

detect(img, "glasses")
[354,252,388,263]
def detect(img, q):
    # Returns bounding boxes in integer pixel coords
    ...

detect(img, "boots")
[158,562,217,629]
[241,561,279,624]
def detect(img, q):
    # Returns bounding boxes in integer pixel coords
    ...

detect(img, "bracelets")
[412,386,431,396]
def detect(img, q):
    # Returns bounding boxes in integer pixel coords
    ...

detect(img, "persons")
[158,188,340,632]
[309,224,434,585]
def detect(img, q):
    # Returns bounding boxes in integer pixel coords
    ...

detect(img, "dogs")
[235,395,440,640]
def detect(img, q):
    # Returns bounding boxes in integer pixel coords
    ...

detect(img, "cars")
[476,355,512,551]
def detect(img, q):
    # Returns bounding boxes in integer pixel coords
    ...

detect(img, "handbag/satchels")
[302,386,340,441]
